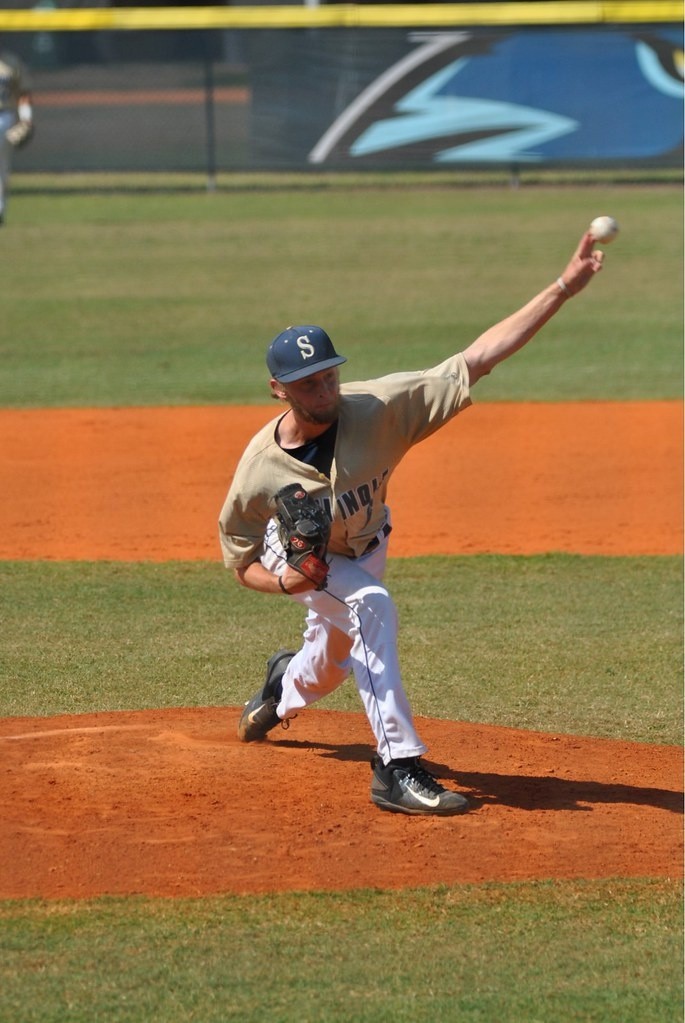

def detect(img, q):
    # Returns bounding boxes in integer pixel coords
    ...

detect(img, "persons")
[0,49,35,227]
[218,232,603,816]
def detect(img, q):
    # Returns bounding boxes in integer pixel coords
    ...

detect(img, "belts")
[362,523,393,556]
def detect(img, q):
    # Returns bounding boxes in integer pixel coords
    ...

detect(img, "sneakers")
[238,652,298,742]
[370,756,469,816]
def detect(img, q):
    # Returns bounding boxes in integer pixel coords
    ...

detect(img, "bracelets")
[558,278,572,297]
[279,576,291,595]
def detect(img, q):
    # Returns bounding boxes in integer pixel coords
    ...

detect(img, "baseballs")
[589,215,619,245]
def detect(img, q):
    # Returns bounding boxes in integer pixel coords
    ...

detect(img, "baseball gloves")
[265,481,335,594]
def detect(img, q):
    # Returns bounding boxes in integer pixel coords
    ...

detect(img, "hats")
[265,325,347,384]
